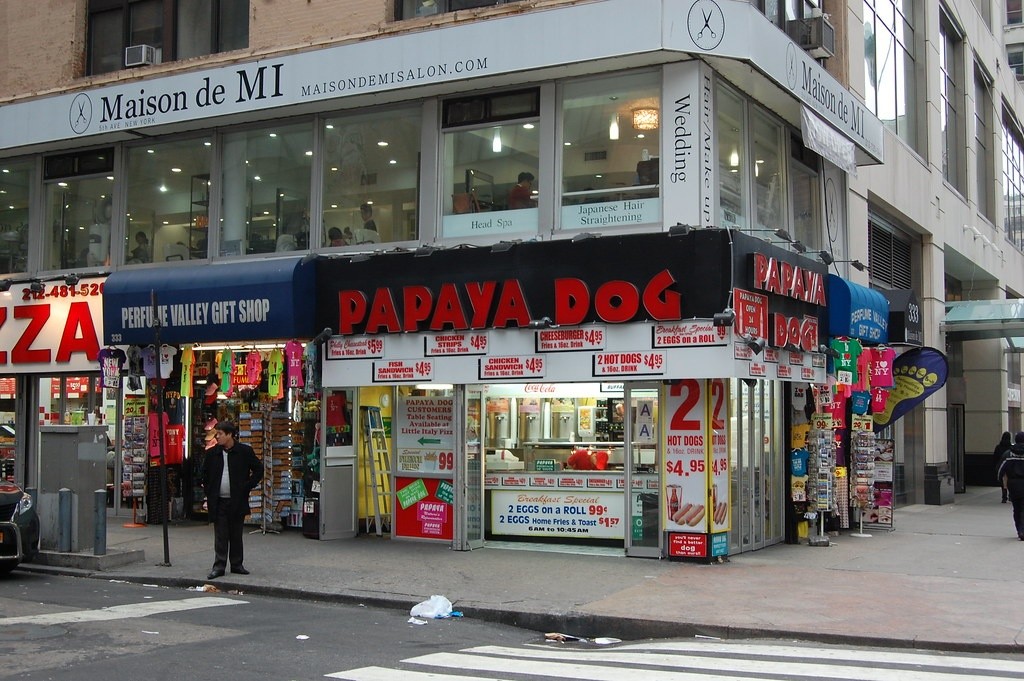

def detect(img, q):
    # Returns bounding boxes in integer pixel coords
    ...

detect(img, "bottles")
[670,489,678,521]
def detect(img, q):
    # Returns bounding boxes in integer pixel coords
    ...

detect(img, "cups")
[69,411,84,426]
[88,414,96,425]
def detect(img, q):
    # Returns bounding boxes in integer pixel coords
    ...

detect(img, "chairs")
[0,229,24,273]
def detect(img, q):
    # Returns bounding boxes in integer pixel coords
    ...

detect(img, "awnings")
[102,254,316,346]
[823,272,890,344]
[860,288,923,348]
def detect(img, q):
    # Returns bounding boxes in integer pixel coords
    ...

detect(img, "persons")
[508,172,536,210]
[275,203,377,251]
[994,432,1014,503]
[999,431,1024,541]
[202,421,264,579]
[131,231,153,263]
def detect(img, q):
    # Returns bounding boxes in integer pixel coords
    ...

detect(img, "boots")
[1002,488,1008,503]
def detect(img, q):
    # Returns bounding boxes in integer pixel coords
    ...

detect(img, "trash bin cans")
[640,493,658,547]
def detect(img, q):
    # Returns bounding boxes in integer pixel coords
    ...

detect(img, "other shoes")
[1018,534,1024,541]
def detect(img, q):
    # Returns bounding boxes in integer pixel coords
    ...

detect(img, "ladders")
[360,405,392,538]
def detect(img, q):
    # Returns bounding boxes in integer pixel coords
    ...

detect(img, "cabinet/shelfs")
[189,173,209,260]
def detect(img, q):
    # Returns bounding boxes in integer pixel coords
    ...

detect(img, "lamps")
[492,126,502,152]
[607,109,659,140]
[300,233,601,267]
[738,229,864,271]
[965,224,1008,263]
[528,317,561,330]
[66,272,79,285]
[32,278,43,292]
[714,309,840,358]
[668,222,703,237]
[0,278,12,292]
[313,327,333,344]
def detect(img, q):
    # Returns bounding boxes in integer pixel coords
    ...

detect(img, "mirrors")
[465,169,495,211]
[278,188,308,236]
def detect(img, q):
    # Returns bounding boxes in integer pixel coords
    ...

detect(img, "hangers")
[109,343,167,348]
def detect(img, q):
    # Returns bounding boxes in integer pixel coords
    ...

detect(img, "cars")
[0,479,40,577]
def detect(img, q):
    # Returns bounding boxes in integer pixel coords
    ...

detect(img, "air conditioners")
[801,16,836,58]
[126,45,157,67]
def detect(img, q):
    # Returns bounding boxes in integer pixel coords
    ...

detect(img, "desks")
[530,184,659,200]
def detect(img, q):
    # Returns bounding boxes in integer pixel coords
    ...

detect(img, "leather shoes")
[230,566,249,574]
[208,570,225,579]
